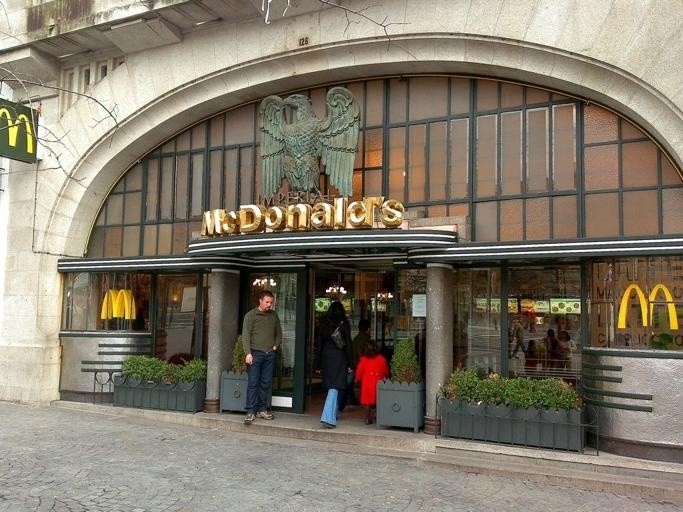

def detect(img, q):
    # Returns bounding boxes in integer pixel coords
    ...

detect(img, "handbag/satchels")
[331,320,346,350]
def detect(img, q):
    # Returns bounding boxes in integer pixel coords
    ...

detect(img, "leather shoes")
[322,422,336,429]
[366,416,376,425]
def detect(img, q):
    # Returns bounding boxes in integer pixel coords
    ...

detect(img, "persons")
[346,319,372,404]
[241,291,282,424]
[508,317,577,376]
[354,339,391,424]
[315,302,354,429]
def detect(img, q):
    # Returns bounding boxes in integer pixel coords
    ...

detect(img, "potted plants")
[220,335,249,415]
[113,355,205,413]
[376,339,425,434]
[439,367,584,455]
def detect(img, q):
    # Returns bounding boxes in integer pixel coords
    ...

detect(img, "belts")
[250,348,274,355]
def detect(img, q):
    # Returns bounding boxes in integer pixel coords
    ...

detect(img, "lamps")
[377,289,394,303]
[323,282,348,300]
[253,275,277,287]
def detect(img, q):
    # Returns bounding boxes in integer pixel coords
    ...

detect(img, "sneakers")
[256,410,275,420]
[244,412,256,425]
